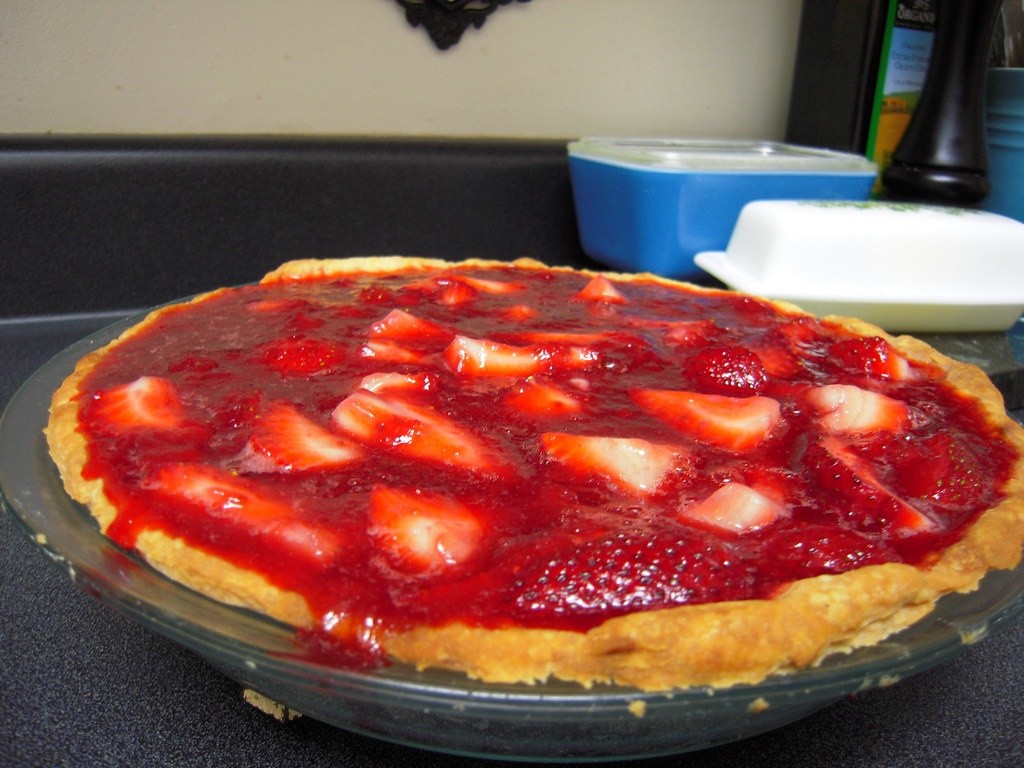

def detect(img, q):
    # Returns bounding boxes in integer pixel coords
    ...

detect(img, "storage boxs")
[566,136,880,281]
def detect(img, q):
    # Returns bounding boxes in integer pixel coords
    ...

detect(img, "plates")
[0,299,1024,765]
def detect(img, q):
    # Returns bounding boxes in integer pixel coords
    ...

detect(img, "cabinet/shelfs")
[781,0,934,201]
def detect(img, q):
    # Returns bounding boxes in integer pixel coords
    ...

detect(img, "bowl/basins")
[694,196,1024,334]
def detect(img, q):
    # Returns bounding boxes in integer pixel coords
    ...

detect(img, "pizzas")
[43,255,1024,691]
